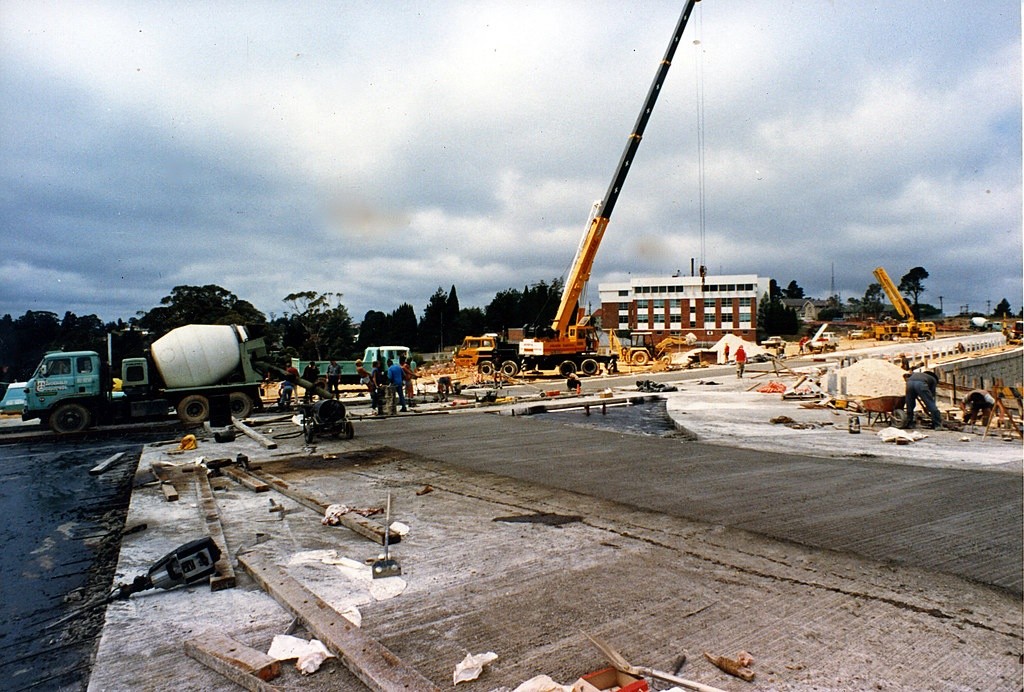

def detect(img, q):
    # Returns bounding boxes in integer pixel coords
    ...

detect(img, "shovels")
[372,491,403,579]
[581,627,727,692]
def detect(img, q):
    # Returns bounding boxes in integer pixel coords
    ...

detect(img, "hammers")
[652,654,686,690]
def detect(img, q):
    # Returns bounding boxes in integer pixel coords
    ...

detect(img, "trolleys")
[846,395,908,430]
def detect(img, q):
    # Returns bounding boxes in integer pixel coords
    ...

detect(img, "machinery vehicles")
[20,323,267,435]
[455,0,702,379]
[625,331,698,367]
[969,317,1002,332]
[1000,312,1023,345]
[873,266,936,340]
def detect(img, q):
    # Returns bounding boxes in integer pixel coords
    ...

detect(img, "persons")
[905,371,949,432]
[734,345,747,379]
[286,362,300,405]
[438,376,455,402]
[274,381,294,413]
[567,373,581,395]
[798,338,804,354]
[490,356,503,390]
[301,361,320,404]
[900,352,909,371]
[356,356,422,415]
[961,389,996,426]
[723,343,729,364]
[326,359,341,401]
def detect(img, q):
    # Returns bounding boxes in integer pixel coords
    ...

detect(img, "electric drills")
[44,536,223,630]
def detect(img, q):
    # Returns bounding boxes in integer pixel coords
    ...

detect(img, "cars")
[761,336,787,349]
[823,332,839,346]
[851,330,863,339]
